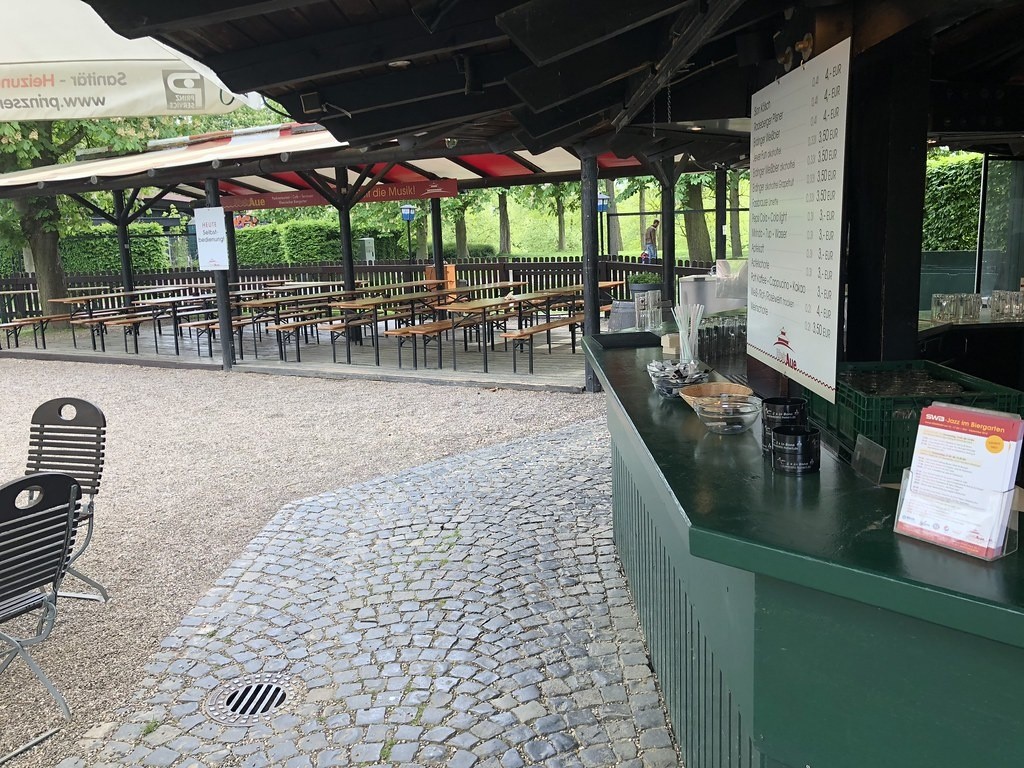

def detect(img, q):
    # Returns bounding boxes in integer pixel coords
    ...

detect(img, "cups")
[931,290,1023,325]
[632,289,664,333]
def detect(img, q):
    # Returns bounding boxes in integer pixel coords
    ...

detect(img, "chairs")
[24,398,111,603]
[0,472,82,766]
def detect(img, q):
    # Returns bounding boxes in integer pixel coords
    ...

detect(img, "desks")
[534,281,625,333]
[262,280,369,308]
[47,286,189,351]
[189,279,293,294]
[328,289,471,367]
[130,289,273,356]
[434,292,562,374]
[443,281,530,343]
[355,279,453,338]
[239,289,366,361]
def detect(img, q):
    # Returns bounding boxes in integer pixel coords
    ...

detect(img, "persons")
[644,219,659,263]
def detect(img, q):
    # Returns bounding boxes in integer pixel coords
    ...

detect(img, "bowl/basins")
[645,360,709,401]
[678,382,754,415]
[693,393,762,434]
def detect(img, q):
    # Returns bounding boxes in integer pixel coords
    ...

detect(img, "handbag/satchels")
[640,249,649,261]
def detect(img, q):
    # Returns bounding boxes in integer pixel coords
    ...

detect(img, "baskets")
[679,382,753,418]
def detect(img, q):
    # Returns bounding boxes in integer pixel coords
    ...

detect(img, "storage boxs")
[800,358,1024,484]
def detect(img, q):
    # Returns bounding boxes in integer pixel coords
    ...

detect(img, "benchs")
[0,288,613,376]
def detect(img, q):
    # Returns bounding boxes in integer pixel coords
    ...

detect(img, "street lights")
[597,194,610,260]
[401,204,417,264]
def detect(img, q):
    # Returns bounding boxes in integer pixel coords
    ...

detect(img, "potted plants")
[627,272,665,301]
